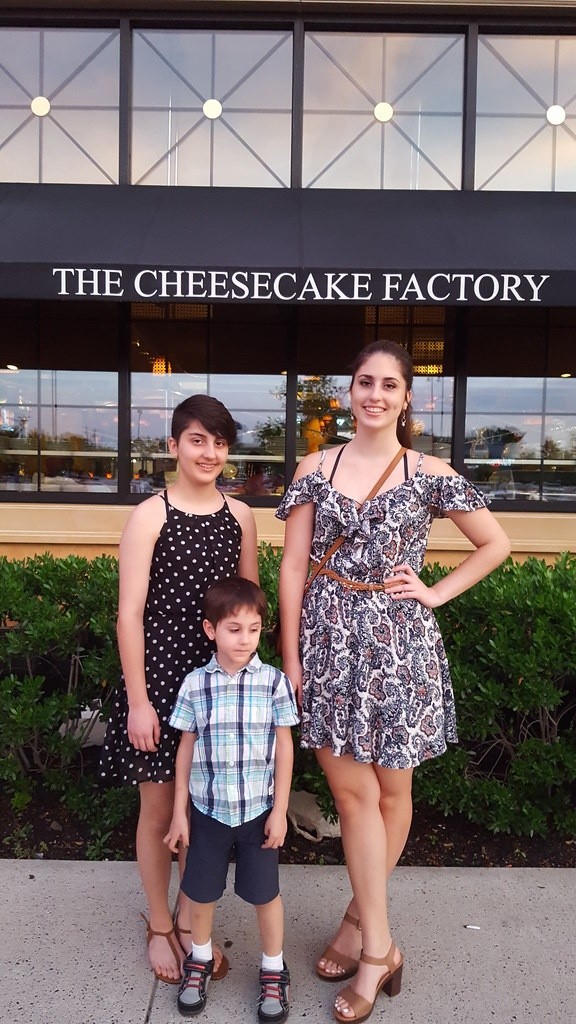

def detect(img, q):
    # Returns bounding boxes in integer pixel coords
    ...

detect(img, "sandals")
[172,914,228,980]
[139,911,189,984]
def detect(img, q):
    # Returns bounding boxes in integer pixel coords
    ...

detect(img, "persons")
[163,579,298,1024]
[115,394,261,984]
[278,339,511,1024]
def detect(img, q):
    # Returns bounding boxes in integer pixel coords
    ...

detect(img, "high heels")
[316,912,364,982]
[333,940,404,1024]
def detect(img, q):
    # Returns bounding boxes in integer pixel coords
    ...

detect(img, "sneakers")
[177,955,214,1016]
[256,959,290,1024]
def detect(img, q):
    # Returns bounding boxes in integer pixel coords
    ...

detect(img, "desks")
[0,482,151,492]
